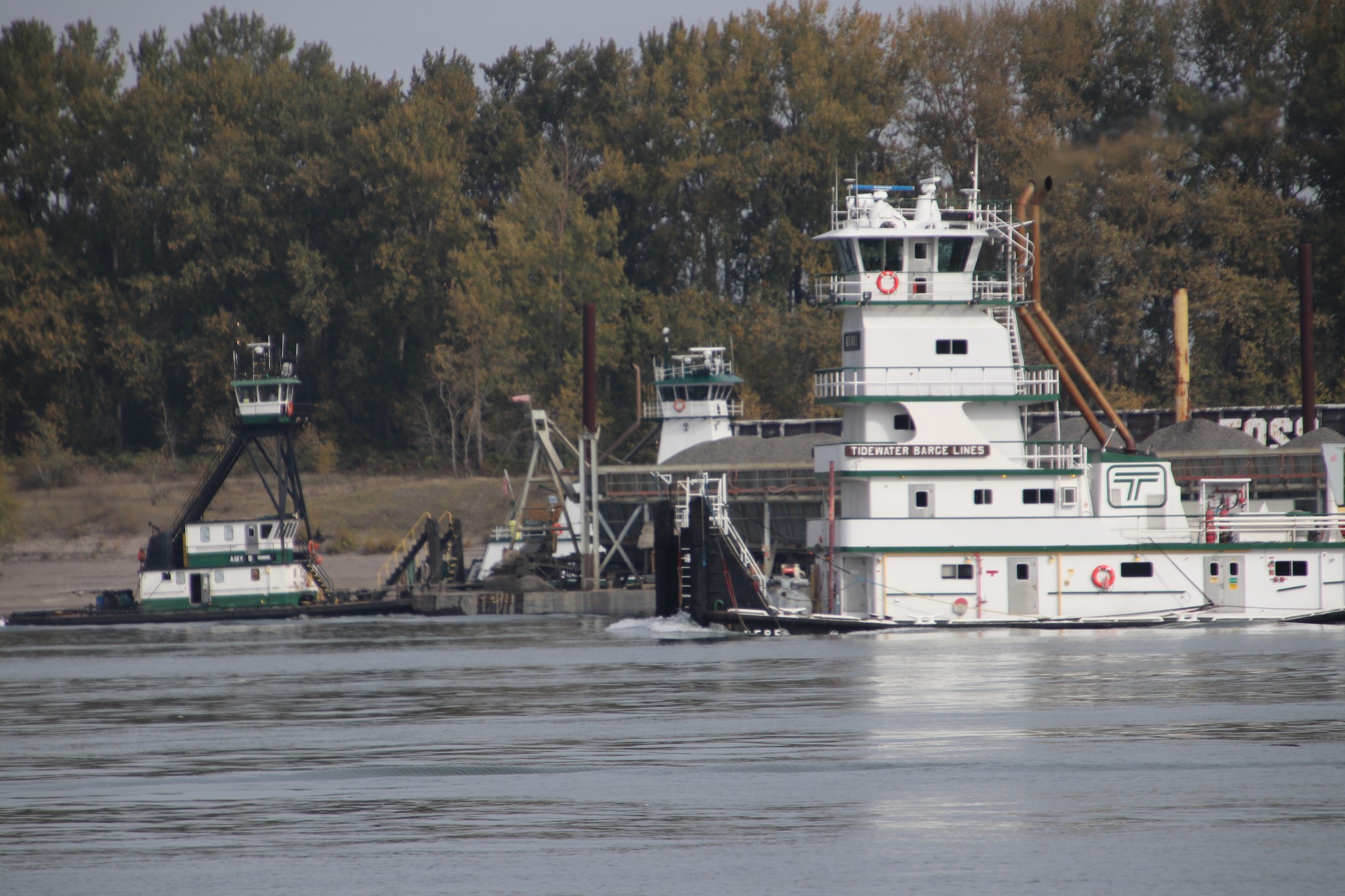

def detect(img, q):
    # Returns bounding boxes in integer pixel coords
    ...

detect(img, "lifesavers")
[1091,565,1115,589]
[286,401,293,417]
[875,272,900,295]
[308,539,315,559]
[673,398,685,412]
[550,523,563,536]
[137,548,146,563]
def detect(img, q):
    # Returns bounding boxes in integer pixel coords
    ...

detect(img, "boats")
[473,326,841,581]
[648,137,1344,631]
